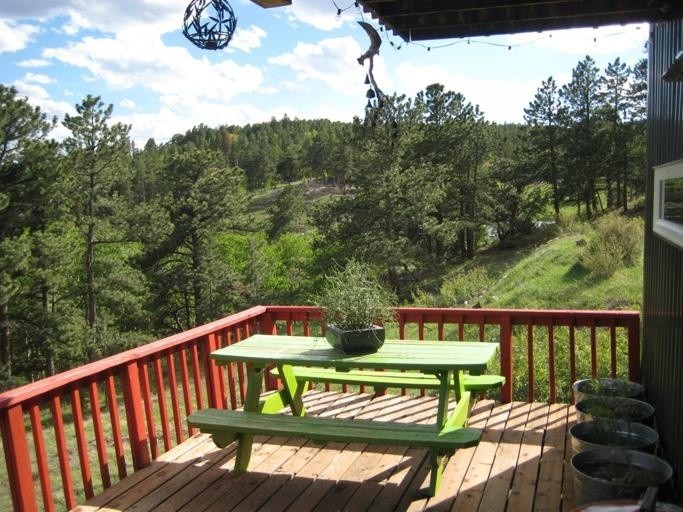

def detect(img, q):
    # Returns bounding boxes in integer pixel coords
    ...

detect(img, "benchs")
[270,365,506,417]
[187,407,482,496]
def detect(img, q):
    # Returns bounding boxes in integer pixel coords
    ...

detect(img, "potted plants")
[569,403,660,452]
[573,371,644,404]
[313,257,399,355]
[570,428,674,506]
[575,378,655,424]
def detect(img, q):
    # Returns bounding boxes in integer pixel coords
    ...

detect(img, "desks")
[209,333,501,496]
